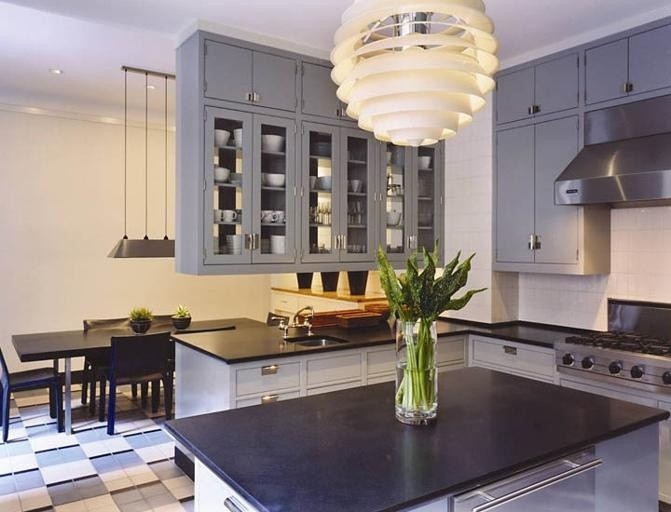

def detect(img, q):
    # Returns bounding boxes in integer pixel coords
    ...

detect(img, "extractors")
[551,94,671,208]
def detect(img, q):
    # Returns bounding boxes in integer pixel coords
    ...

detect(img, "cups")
[348,244,366,253]
[391,184,396,195]
[223,208,237,223]
[275,210,285,225]
[348,176,363,193]
[386,152,393,164]
[396,185,401,195]
[349,201,366,224]
[213,209,226,223]
[263,210,279,224]
[387,186,391,195]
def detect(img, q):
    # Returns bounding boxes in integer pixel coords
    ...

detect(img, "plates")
[226,234,241,254]
[269,235,285,254]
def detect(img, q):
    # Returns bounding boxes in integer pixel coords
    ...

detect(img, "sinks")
[283,335,349,347]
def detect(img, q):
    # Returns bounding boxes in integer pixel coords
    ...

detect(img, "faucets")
[293,305,314,336]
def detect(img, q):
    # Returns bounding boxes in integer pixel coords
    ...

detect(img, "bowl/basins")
[387,211,402,226]
[264,172,285,187]
[262,133,285,152]
[215,168,231,182]
[312,141,329,156]
[233,128,243,148]
[418,156,431,169]
[214,129,230,147]
[310,176,331,191]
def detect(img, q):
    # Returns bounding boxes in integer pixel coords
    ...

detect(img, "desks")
[164,366,671,512]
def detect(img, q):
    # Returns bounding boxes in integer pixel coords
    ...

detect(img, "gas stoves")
[553,328,671,395]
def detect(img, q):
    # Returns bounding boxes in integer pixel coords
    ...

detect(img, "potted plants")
[128,305,153,336]
[375,238,490,426]
[171,303,191,330]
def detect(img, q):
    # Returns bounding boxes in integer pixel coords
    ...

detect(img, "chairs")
[140,314,174,406]
[96,330,171,435]
[173,324,236,336]
[81,318,138,411]
[0,346,64,443]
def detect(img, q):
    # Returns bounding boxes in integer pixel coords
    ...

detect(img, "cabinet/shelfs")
[174,342,303,482]
[177,32,300,276]
[437,334,468,373]
[469,335,559,385]
[583,15,670,113]
[379,138,446,272]
[299,53,378,273]
[300,293,386,318]
[365,344,397,390]
[492,36,613,278]
[269,289,298,321]
[305,346,366,398]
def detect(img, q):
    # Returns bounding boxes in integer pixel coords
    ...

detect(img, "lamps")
[331,0,498,147]
[107,65,178,259]
